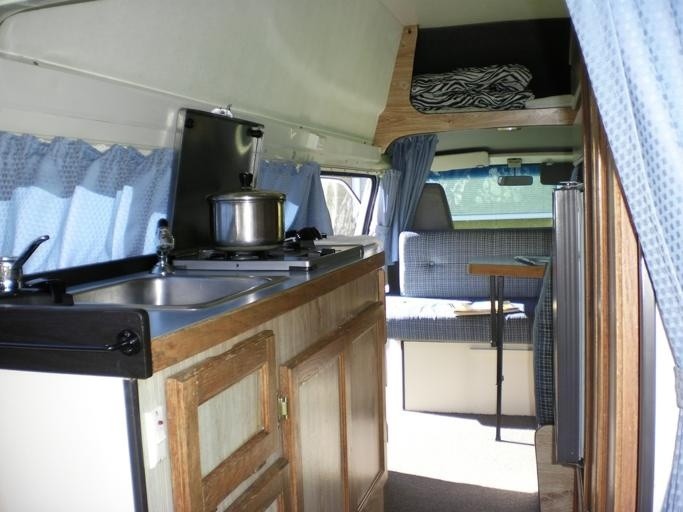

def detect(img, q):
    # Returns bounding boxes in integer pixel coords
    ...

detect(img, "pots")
[0,235,49,280]
[203,169,288,252]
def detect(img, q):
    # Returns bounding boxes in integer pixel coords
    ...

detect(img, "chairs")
[411,183,453,230]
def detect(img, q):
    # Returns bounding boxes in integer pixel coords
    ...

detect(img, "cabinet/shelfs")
[277,300,389,512]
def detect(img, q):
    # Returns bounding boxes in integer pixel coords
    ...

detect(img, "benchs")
[377,229,556,415]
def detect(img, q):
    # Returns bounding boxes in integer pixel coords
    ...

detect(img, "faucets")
[0,230,49,295]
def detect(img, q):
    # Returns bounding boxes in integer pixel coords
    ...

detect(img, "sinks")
[66,266,292,312]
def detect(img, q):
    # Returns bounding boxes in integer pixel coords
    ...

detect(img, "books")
[447,299,519,316]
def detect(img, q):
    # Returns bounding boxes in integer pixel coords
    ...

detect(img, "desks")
[466,255,553,439]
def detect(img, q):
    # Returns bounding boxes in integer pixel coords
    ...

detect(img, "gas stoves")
[170,243,364,273]
